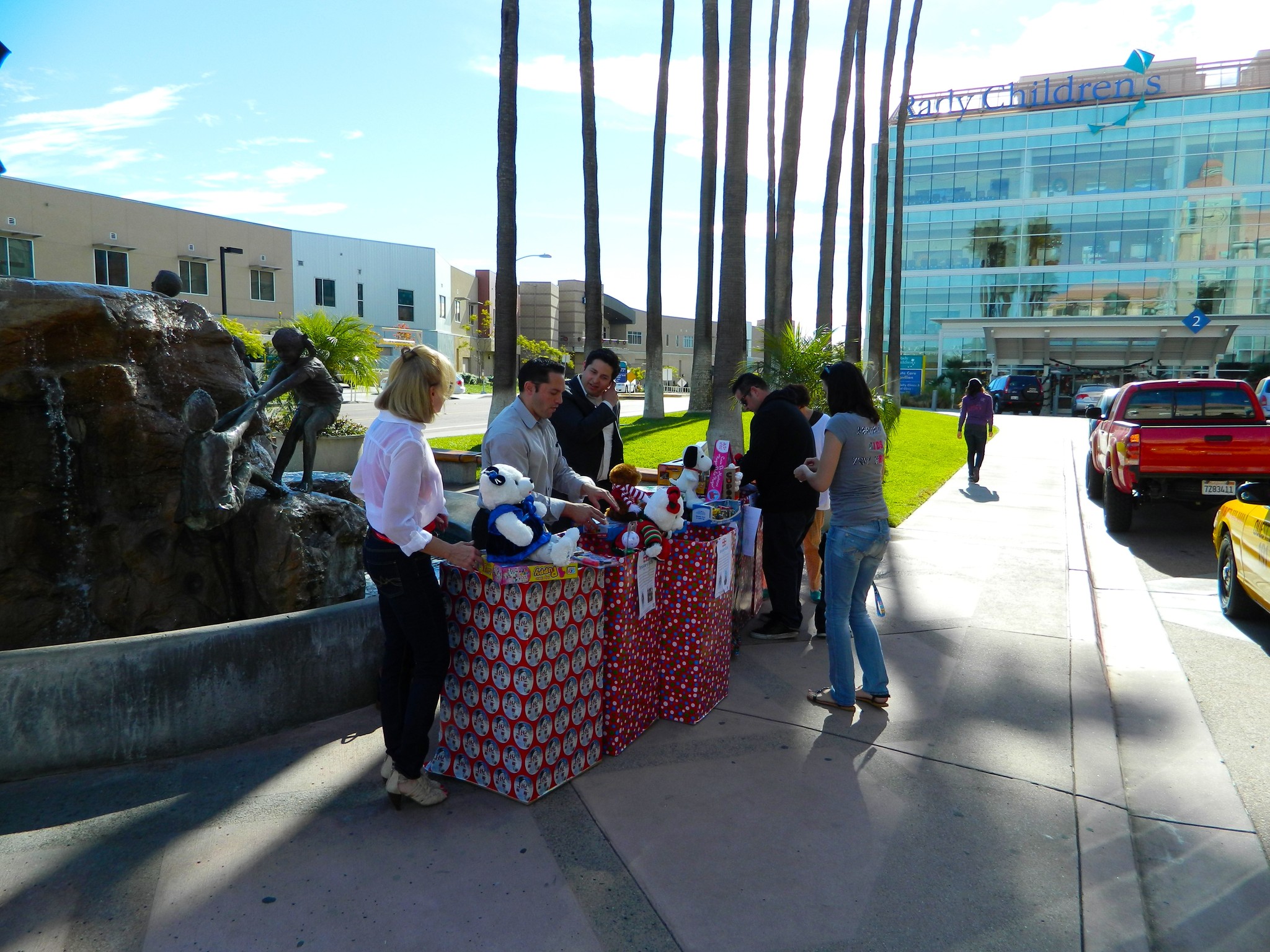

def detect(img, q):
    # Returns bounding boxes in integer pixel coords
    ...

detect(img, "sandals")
[807,687,855,712]
[855,685,891,707]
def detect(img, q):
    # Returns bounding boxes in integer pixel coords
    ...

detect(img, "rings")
[800,480,802,482]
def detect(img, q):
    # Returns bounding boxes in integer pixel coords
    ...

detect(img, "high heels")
[384,769,447,811]
[380,754,440,789]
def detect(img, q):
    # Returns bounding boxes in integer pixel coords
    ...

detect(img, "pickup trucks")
[1084,379,1270,534]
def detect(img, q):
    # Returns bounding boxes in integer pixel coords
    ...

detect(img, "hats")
[968,379,980,386]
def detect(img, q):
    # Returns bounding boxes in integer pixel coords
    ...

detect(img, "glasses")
[739,386,760,407]
[822,364,833,374]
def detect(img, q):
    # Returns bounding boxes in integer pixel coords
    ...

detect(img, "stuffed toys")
[669,445,712,509]
[605,462,650,523]
[614,486,685,562]
[472,464,579,567]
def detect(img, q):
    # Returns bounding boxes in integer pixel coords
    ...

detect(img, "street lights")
[219,245,242,314]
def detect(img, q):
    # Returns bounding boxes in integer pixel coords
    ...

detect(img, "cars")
[1085,388,1120,438]
[614,370,637,393]
[1213,481,1270,620]
[1068,384,1114,416]
[1255,377,1270,419]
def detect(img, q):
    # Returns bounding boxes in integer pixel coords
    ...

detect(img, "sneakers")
[750,620,799,640]
[817,629,826,638]
[762,588,769,600]
[810,589,821,603]
[968,475,974,482]
[760,610,800,631]
[973,467,979,482]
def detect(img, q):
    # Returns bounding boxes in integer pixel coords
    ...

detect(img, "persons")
[177,389,289,532]
[794,361,890,711]
[150,269,181,298]
[548,349,623,535]
[957,378,993,483]
[349,343,485,808]
[472,356,618,550]
[733,372,820,640]
[763,384,830,602]
[250,327,343,496]
[666,381,688,392]
[637,382,642,390]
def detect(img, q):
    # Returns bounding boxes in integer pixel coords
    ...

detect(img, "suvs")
[985,375,1043,415]
